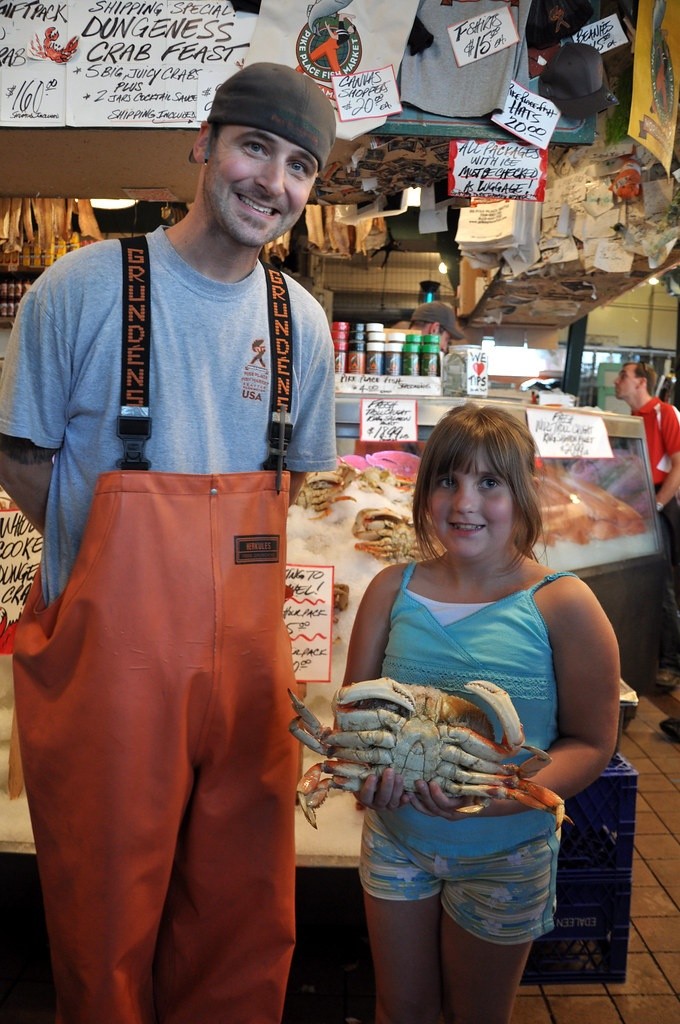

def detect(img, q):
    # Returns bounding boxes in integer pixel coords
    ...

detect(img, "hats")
[410,301,465,340]
[188,63,336,171]
[538,43,621,118]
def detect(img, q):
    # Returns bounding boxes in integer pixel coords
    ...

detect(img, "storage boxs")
[517,754,639,986]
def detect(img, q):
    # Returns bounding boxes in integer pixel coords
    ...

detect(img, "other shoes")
[659,718,680,745]
[654,668,680,686]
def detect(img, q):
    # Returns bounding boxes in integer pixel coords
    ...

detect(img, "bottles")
[418,281,440,306]
[0,299,20,318]
[332,321,440,378]
[0,279,31,299]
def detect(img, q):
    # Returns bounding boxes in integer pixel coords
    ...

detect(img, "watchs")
[656,502,663,511]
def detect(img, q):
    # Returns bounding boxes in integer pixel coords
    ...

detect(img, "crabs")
[287,453,356,525]
[352,504,448,571]
[287,666,576,831]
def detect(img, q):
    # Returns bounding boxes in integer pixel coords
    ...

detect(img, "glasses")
[639,362,646,373]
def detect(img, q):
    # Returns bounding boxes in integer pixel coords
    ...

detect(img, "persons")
[0,61,338,1024]
[390,301,464,353]
[614,361,680,686]
[330,404,621,1024]
